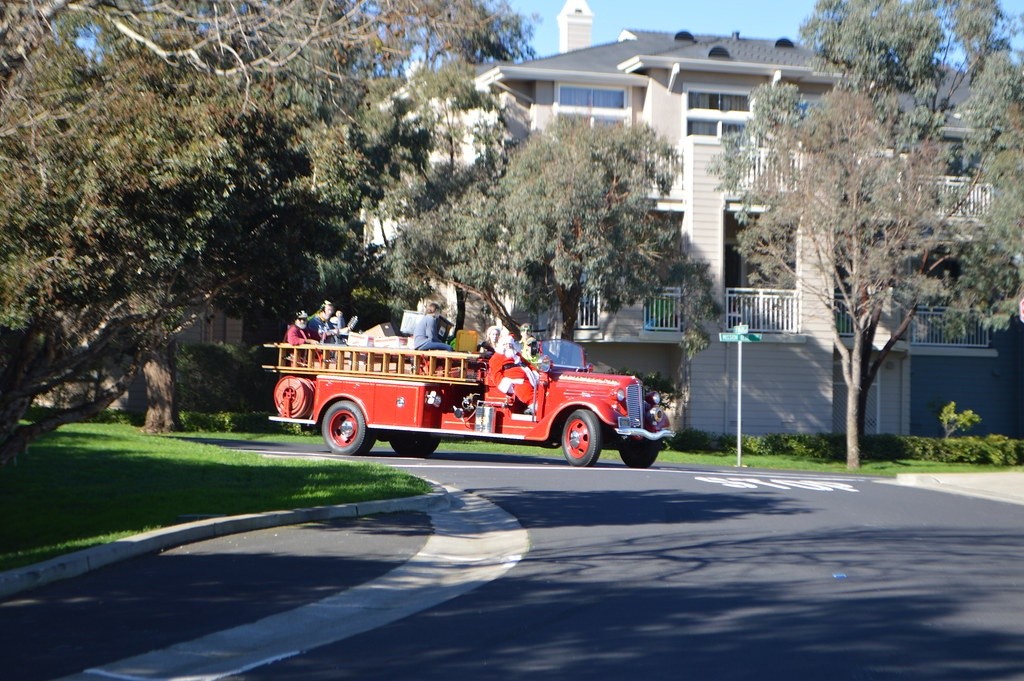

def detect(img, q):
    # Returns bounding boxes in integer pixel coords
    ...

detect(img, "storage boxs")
[348,310,455,365]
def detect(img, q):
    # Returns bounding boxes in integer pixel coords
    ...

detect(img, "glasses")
[297,318,306,321]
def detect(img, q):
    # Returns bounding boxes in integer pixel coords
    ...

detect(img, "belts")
[502,362,526,369]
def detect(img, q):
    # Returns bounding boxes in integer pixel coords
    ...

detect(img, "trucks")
[259,336,677,468]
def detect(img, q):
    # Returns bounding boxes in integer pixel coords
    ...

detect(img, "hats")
[520,323,532,330]
[497,333,516,344]
[295,310,308,319]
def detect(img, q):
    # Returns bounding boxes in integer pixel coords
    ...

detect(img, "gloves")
[507,384,515,394]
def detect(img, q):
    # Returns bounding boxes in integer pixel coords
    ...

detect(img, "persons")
[518,323,538,358]
[283,300,338,367]
[477,327,500,360]
[413,304,454,376]
[488,334,540,415]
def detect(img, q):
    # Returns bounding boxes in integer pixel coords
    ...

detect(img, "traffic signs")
[719,333,763,342]
[733,324,750,335]
